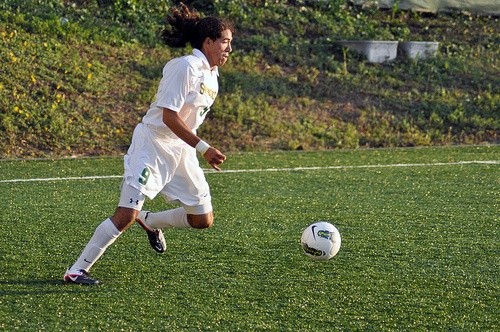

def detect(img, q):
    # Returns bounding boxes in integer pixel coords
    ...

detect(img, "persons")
[61,0,234,287]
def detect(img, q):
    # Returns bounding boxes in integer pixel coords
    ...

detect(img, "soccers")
[301,222,341,259]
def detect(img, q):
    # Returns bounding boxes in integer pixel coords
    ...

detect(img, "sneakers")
[136,211,167,253]
[63,267,100,284]
